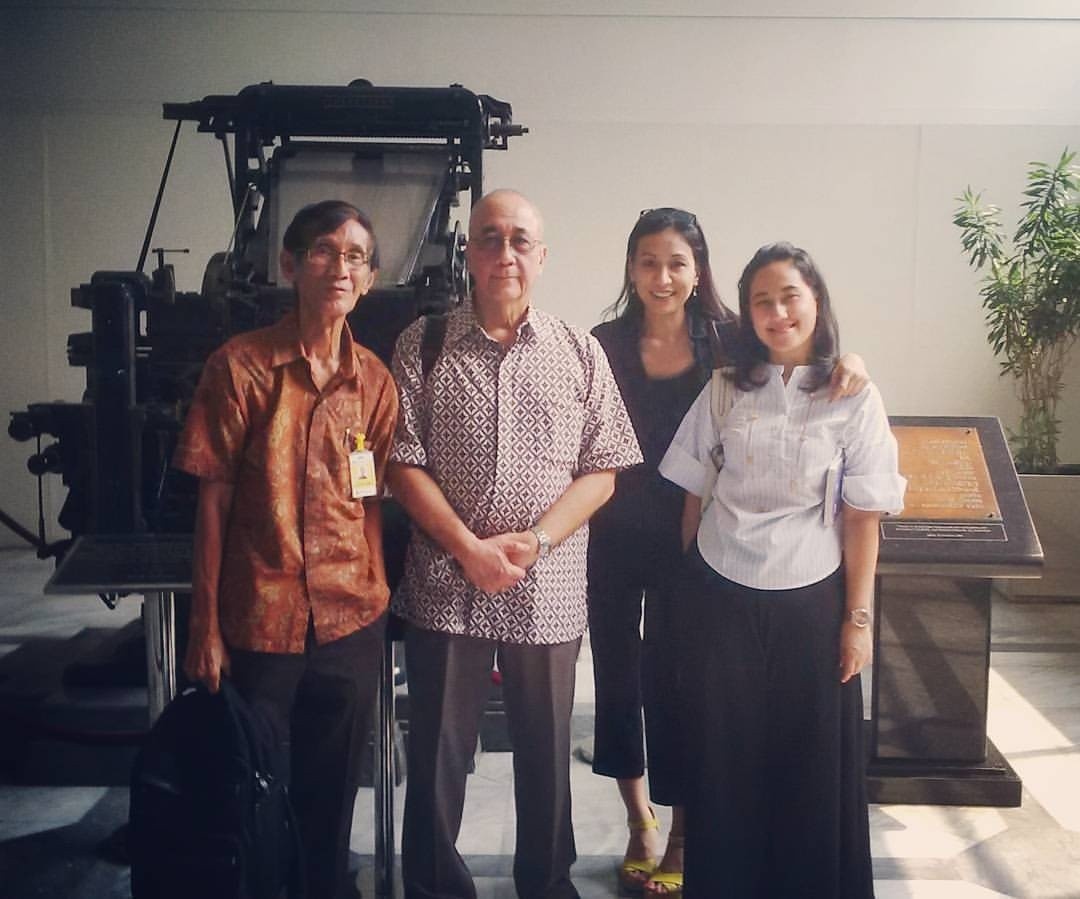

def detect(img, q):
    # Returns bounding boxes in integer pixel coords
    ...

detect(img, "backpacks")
[128,676,300,899]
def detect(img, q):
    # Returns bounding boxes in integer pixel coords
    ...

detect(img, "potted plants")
[948,152,1079,603]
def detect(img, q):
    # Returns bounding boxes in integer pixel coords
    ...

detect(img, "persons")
[387,188,645,899]
[656,238,909,899]
[590,206,870,898]
[166,198,399,898]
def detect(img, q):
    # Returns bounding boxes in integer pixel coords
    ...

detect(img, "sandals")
[622,804,661,891]
[642,833,684,899]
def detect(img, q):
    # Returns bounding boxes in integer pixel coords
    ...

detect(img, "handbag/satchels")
[376,495,414,643]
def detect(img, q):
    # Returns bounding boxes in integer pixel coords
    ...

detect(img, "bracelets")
[528,525,557,561]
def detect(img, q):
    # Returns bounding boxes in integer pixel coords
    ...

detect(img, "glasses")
[639,208,696,226]
[302,240,374,271]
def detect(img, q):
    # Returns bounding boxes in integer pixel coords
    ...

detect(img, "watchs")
[845,607,874,630]
